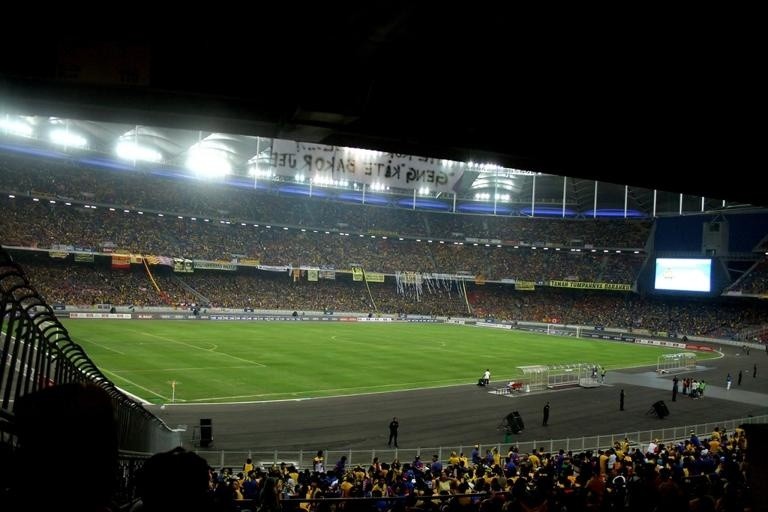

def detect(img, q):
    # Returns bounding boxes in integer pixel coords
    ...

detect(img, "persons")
[752,364,757,377]
[765,344,768,355]
[746,346,750,355]
[672,374,678,392]
[742,345,746,352]
[737,369,742,384]
[482,368,490,385]
[598,365,608,384]
[591,361,597,377]
[725,372,732,390]
